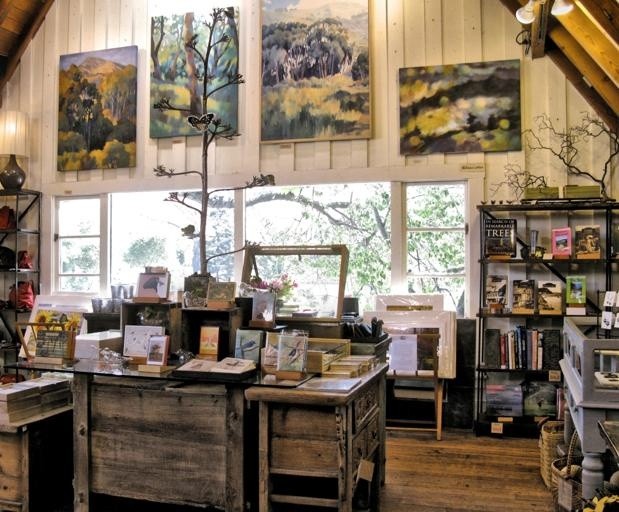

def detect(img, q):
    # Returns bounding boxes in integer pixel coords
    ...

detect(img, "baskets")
[550,425,584,512]
[539,419,577,490]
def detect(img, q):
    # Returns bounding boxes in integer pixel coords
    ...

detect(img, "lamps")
[0,110,31,190]
[515,0,574,24]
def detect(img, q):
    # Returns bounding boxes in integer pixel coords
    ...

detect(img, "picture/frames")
[257,1,374,142]
[363,293,459,380]
[565,276,587,304]
[551,228,572,256]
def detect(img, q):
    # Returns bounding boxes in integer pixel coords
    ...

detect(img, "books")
[174,290,382,392]
[146,334,171,366]
[1,374,72,425]
[484,212,602,418]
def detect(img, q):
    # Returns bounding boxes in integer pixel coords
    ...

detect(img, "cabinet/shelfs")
[475,201,617,435]
[0,189,44,376]
[3,342,391,512]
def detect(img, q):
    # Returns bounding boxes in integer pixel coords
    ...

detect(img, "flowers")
[241,271,298,303]
